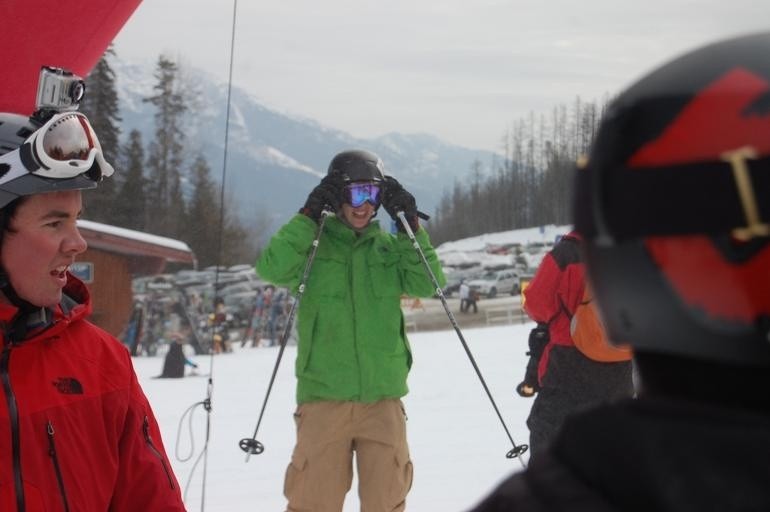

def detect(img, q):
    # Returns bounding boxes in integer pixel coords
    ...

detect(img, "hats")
[0,112,97,209]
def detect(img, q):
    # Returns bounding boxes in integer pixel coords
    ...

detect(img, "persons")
[0,106,189,511]
[253,147,448,512]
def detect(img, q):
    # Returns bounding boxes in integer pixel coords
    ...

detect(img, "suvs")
[471,269,521,299]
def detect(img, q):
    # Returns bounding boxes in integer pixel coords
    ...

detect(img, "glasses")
[343,184,381,208]
[0,111,114,186]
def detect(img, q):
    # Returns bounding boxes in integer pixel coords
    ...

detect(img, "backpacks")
[561,231,632,363]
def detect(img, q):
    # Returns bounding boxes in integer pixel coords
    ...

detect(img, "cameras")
[35,65,86,111]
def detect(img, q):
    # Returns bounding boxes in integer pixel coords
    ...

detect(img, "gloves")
[299,177,342,223]
[382,175,430,234]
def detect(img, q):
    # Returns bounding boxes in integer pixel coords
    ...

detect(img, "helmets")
[575,32,770,357]
[328,149,385,186]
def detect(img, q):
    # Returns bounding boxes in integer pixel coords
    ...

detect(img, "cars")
[135,264,291,326]
